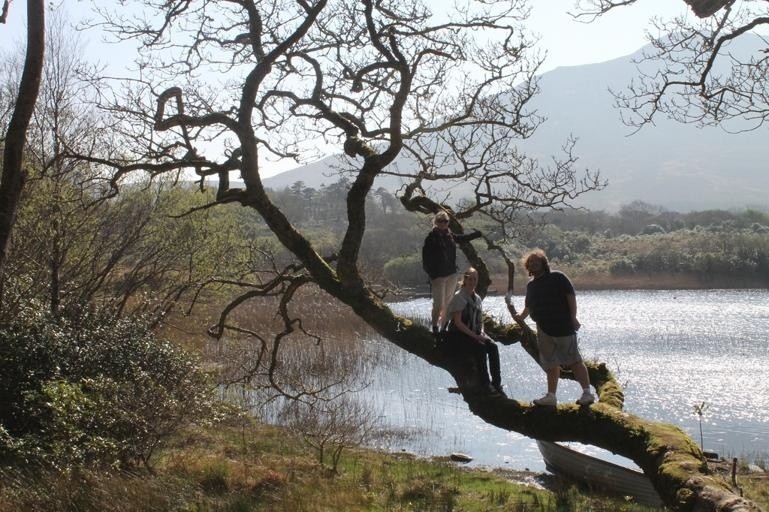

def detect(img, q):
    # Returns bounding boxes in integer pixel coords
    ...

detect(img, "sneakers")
[484,382,508,399]
[532,394,557,406]
[577,394,595,404]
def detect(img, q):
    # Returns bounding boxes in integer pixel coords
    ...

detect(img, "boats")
[534,437,665,508]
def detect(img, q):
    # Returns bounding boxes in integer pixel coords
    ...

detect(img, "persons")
[422,211,458,335]
[446,268,510,398]
[513,250,600,406]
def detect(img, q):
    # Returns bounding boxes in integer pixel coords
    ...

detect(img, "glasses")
[438,218,449,223]
